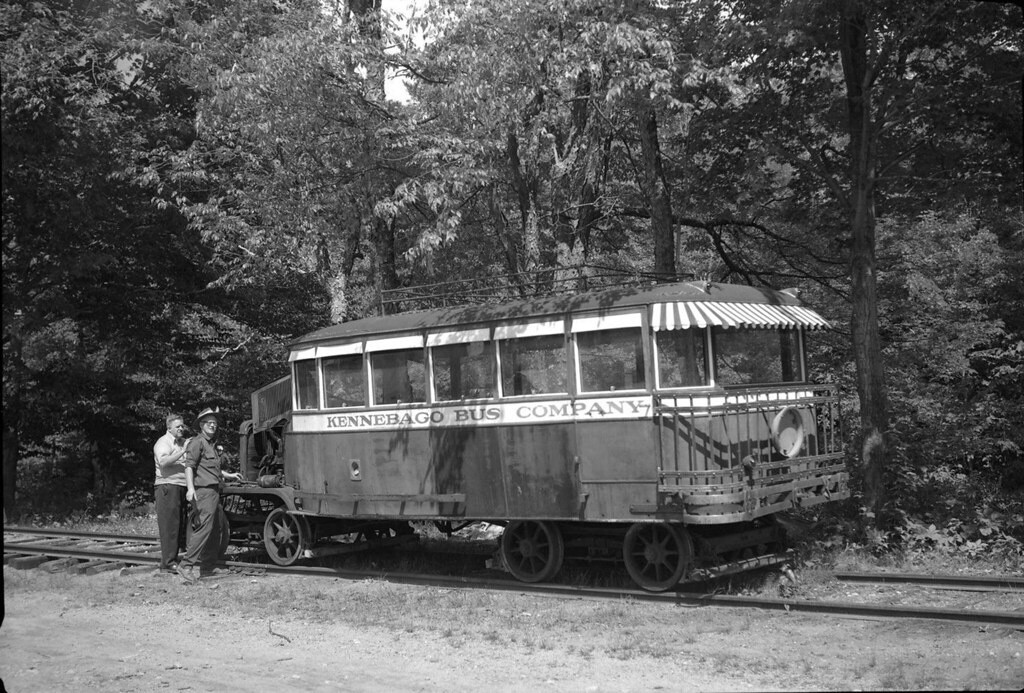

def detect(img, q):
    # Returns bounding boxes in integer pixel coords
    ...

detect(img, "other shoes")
[176,565,197,582]
[199,574,219,580]
[159,564,180,573]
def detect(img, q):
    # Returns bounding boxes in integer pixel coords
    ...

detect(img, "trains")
[221,270,854,593]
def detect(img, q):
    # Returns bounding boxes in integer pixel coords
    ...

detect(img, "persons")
[153,414,194,574]
[176,408,244,582]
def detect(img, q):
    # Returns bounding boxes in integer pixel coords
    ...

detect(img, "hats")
[197,407,218,419]
